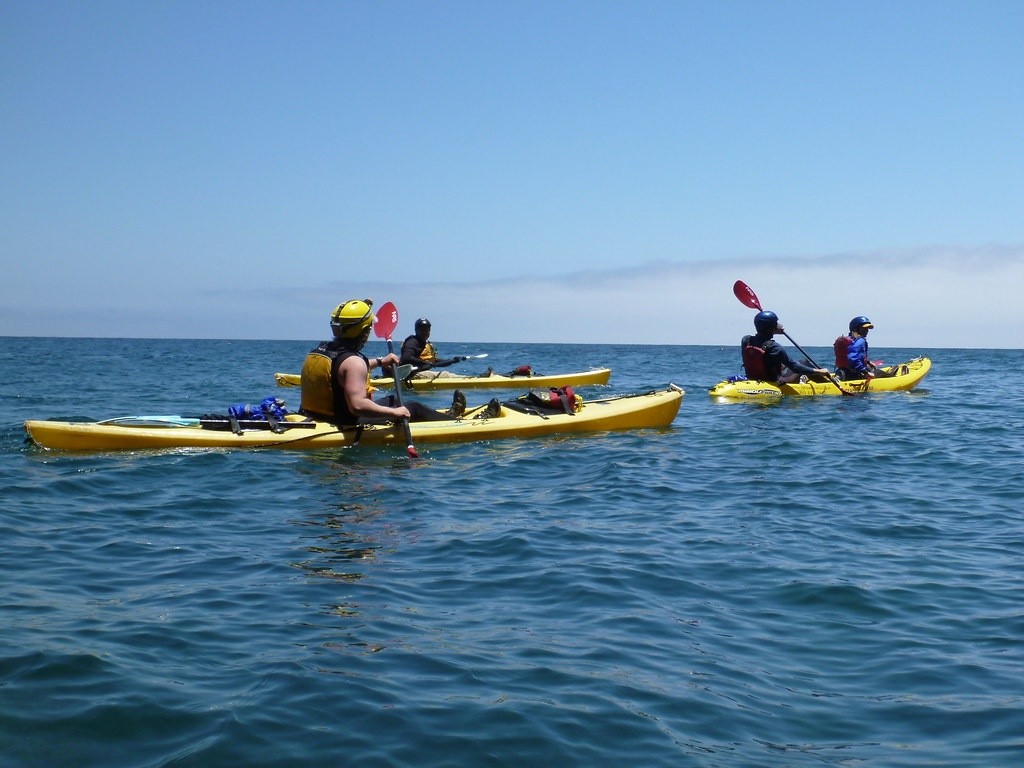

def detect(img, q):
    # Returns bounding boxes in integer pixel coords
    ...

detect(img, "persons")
[399,318,493,378]
[299,303,500,423]
[740,311,846,383]
[840,316,908,378]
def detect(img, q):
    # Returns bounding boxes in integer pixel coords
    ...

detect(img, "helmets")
[415,318,432,329]
[754,310,779,326]
[329,299,378,338]
[849,316,872,332]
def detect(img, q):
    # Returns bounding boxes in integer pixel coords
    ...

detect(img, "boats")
[22,384,684,453]
[706,355,932,399]
[275,366,611,392]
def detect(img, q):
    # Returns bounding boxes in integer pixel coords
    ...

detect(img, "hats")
[861,323,874,330]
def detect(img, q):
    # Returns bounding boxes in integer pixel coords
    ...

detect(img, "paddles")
[418,354,487,371]
[94,414,316,435]
[373,301,419,459]
[861,359,883,392]
[732,280,855,396]
